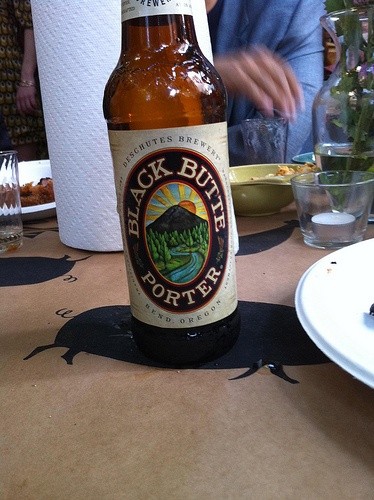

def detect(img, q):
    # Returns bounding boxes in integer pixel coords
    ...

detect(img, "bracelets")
[19,80,35,87]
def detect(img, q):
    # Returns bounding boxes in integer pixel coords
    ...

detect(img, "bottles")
[313,8,374,173]
[102,1,240,369]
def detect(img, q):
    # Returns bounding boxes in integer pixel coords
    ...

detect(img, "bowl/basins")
[291,152,316,165]
[228,162,321,216]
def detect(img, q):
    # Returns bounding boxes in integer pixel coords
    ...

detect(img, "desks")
[0,192,374,500]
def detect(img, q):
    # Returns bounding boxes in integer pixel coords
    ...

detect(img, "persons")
[205,0,325,168]
[0,0,49,162]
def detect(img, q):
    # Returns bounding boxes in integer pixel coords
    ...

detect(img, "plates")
[295,236,374,393]
[0,160,57,221]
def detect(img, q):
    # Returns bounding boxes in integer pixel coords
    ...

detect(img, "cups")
[289,170,374,249]
[0,150,24,256]
[312,212,355,246]
